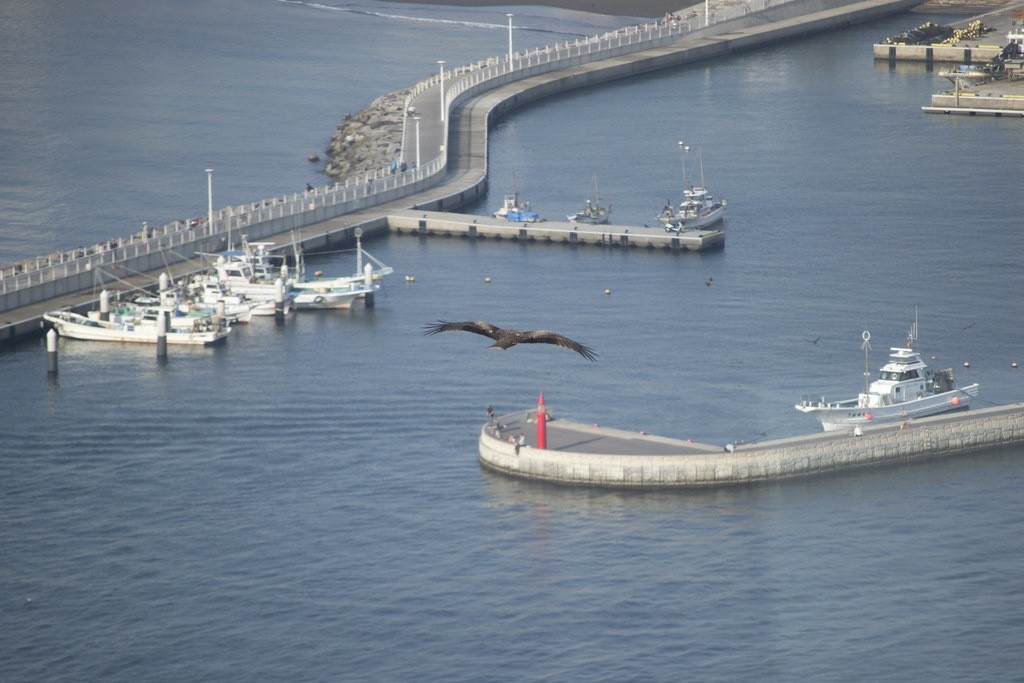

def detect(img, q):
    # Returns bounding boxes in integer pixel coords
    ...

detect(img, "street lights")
[205,169,215,234]
[438,61,446,121]
[413,117,421,179]
[506,14,514,72]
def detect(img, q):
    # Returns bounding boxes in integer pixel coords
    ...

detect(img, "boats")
[795,306,979,432]
[86,283,259,327]
[42,268,232,345]
[493,193,546,222]
[937,64,1003,88]
[565,171,613,224]
[177,217,393,315]
[653,144,728,229]
